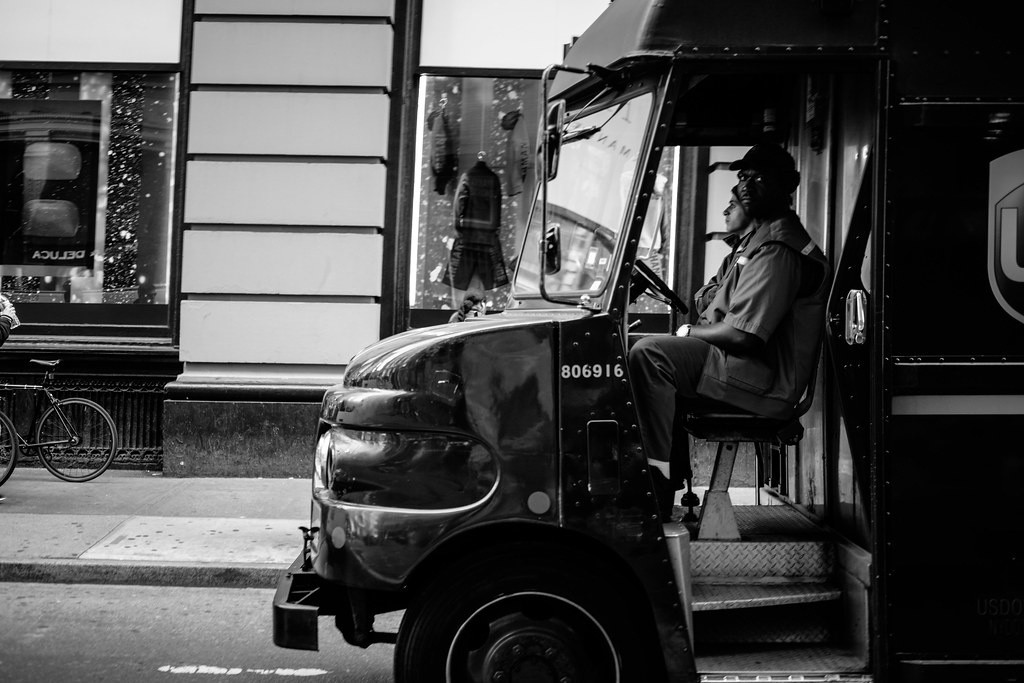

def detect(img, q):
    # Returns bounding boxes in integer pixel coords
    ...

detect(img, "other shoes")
[648,464,675,514]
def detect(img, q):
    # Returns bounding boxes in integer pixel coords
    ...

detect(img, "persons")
[628,141,834,482]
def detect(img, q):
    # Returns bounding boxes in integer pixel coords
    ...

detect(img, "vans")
[273,0,1021,683]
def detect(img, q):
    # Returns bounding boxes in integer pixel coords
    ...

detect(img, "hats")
[728,141,800,191]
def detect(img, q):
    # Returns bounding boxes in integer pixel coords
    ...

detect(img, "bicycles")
[0,359,119,489]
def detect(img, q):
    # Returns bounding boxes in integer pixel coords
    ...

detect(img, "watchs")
[676,324,691,337]
